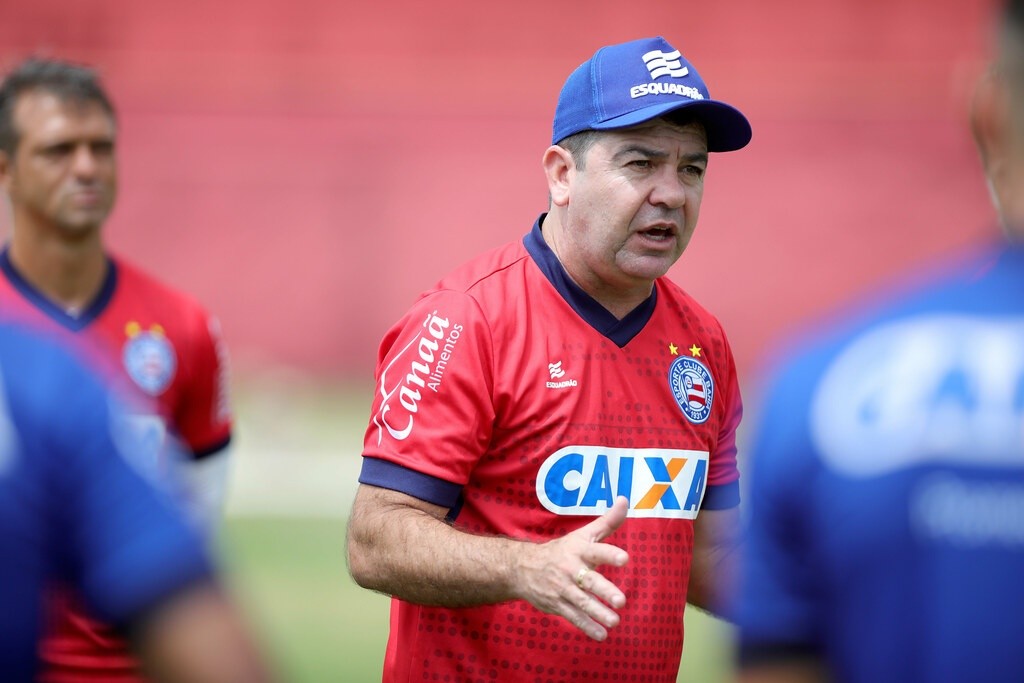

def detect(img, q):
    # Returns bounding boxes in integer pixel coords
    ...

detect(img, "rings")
[575,568,592,589]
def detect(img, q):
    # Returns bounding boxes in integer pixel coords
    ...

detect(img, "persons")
[346,36,745,683]
[0,318,277,683]
[726,0,1024,683]
[0,54,235,683]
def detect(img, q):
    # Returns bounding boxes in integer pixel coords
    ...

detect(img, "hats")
[552,37,752,153]
